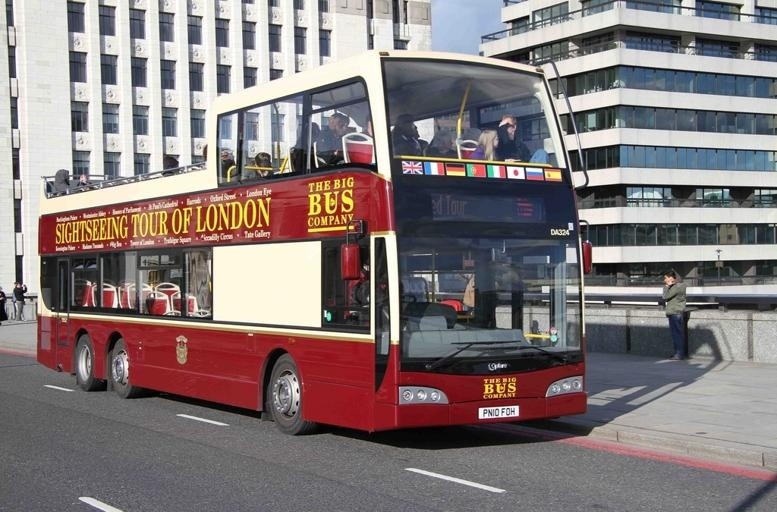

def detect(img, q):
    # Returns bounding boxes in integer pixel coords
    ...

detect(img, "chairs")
[67,277,210,317]
[313,137,329,168]
[455,136,481,160]
[342,132,377,165]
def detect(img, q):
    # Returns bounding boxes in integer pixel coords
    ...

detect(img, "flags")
[544,168,562,182]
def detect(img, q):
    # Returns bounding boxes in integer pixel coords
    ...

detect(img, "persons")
[364,276,389,302]
[55,170,70,192]
[77,174,95,191]
[0,282,27,325]
[298,114,374,169]
[392,113,547,164]
[662,271,686,360]
[220,147,273,179]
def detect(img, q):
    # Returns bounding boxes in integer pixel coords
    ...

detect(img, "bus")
[33,49,594,436]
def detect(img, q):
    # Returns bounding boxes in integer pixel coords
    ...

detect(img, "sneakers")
[665,350,684,360]
